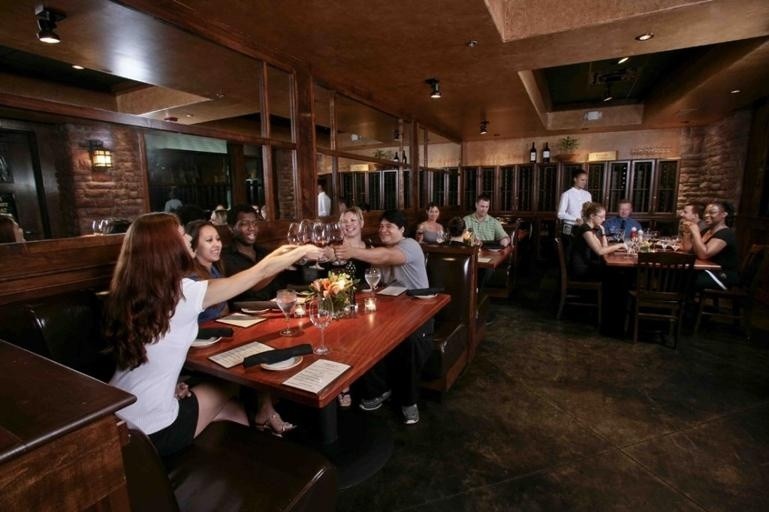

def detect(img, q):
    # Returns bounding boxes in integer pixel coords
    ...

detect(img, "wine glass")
[365,267,381,295]
[283,220,347,270]
[308,294,336,354]
[610,225,683,258]
[275,288,301,338]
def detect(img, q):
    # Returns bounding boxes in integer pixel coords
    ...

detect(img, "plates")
[414,293,440,299]
[193,334,224,348]
[242,306,270,313]
[260,354,304,371]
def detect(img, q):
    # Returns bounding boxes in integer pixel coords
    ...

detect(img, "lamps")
[479,121,490,134]
[424,76,444,102]
[30,5,66,47]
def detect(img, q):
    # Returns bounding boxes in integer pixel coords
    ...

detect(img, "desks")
[189,279,451,468]
[589,228,722,284]
[439,231,513,305]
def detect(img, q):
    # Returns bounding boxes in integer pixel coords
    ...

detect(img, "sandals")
[338,391,351,407]
[254,411,299,434]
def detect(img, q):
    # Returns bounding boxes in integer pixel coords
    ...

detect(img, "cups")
[364,297,376,314]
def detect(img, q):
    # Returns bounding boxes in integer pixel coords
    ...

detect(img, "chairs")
[489,220,534,300]
[700,242,768,338]
[549,237,606,327]
[625,250,695,347]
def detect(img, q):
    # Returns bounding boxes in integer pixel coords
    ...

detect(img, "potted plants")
[556,133,583,165]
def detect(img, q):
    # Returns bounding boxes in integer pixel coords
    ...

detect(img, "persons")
[417,193,510,293]
[0,198,268,242]
[682,201,738,326]
[99,192,335,450]
[595,199,643,237]
[558,168,592,244]
[569,202,629,339]
[676,204,707,241]
[316,205,431,424]
[318,185,331,215]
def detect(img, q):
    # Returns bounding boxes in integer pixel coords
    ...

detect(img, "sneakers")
[359,388,391,411]
[401,400,420,425]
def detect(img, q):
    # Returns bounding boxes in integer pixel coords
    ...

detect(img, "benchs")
[267,230,490,389]
[16,286,330,510]
